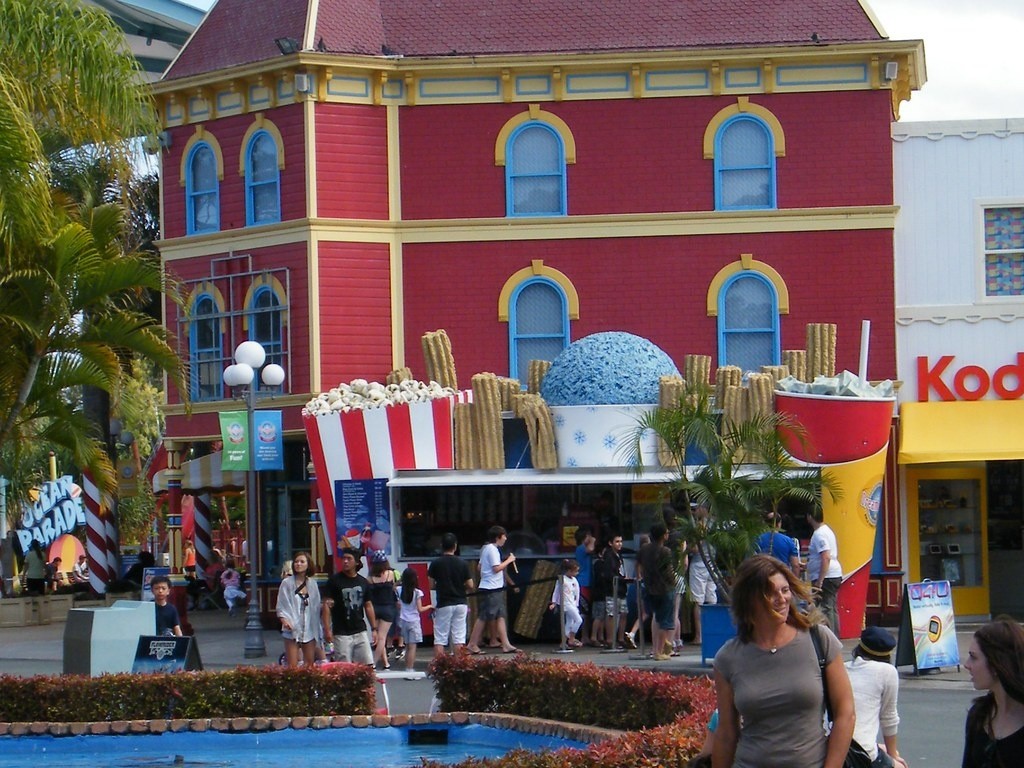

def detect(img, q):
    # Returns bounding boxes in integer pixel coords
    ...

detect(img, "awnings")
[897,401,1024,464]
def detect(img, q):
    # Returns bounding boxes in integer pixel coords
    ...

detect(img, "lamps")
[883,61,897,79]
[294,73,310,90]
[275,36,298,55]
[142,132,171,155]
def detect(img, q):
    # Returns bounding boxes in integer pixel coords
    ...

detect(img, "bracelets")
[372,627,378,631]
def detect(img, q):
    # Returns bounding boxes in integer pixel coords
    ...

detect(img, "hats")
[651,523,667,536]
[343,546,362,559]
[372,550,388,563]
[860,626,898,657]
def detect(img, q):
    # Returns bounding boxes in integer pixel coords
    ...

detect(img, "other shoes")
[383,665,392,672]
[403,669,422,680]
[372,665,377,672]
[560,643,573,649]
[650,639,702,660]
[589,631,638,650]
[568,638,581,647]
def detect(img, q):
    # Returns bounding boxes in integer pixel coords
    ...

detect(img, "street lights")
[222,342,285,659]
[109,420,135,559]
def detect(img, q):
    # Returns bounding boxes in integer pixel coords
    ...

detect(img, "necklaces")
[770,648,777,654]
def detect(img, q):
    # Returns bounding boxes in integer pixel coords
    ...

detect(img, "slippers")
[490,642,503,648]
[503,646,523,653]
[478,642,488,648]
[473,649,486,655]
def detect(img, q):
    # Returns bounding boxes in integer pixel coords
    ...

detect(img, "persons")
[219,560,247,616]
[184,539,195,579]
[276,551,334,667]
[149,577,183,637]
[366,550,397,670]
[185,552,223,611]
[72,555,90,583]
[125,551,155,585]
[752,512,800,578]
[322,548,379,668]
[962,620,1024,768]
[806,503,843,642]
[395,501,719,681]
[46,557,64,592]
[213,534,237,562]
[823,626,908,768]
[23,540,47,624]
[711,554,857,768]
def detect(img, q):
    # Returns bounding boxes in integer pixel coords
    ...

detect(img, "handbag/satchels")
[809,624,872,768]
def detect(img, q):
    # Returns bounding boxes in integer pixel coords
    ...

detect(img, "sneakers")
[386,645,397,660]
[395,643,407,660]
